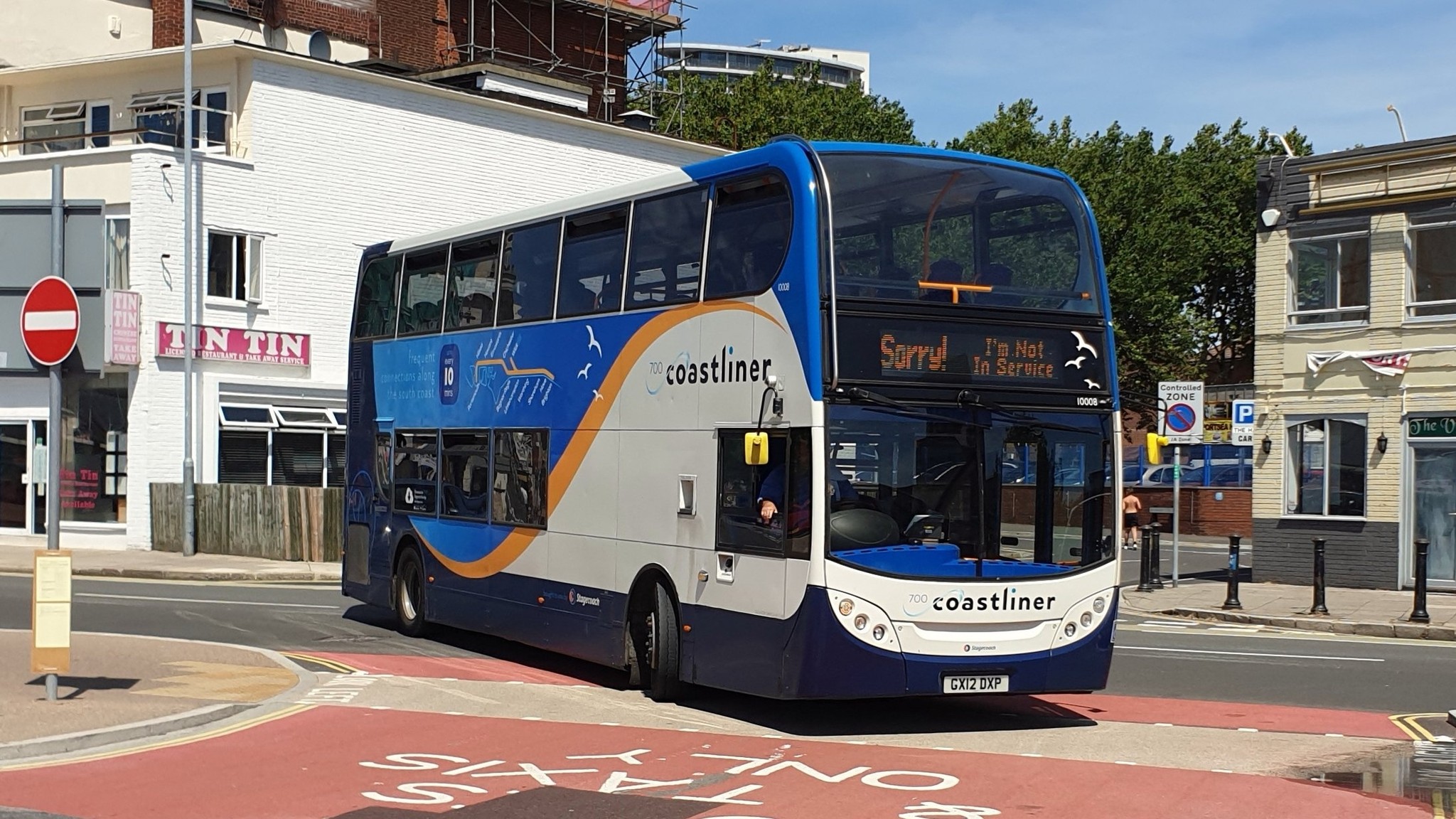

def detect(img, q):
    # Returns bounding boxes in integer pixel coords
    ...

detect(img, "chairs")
[380,295,528,518]
[920,258,1023,306]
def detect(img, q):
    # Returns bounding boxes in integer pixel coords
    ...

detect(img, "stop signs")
[20,276,83,367]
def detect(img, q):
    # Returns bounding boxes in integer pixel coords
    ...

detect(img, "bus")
[342,134,1171,704]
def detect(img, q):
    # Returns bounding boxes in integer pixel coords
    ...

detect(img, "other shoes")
[1123,545,1128,550]
[1132,544,1137,550]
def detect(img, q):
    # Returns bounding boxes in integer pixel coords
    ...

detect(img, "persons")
[757,434,859,520]
[1122,488,1141,550]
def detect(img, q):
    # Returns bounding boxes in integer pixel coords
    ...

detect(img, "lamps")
[1263,435,1272,453]
[247,297,262,306]
[1378,431,1389,452]
[162,164,171,168]
[162,254,170,258]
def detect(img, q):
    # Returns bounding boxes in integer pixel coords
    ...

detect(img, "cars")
[916,440,1253,489]
[1300,473,1364,516]
